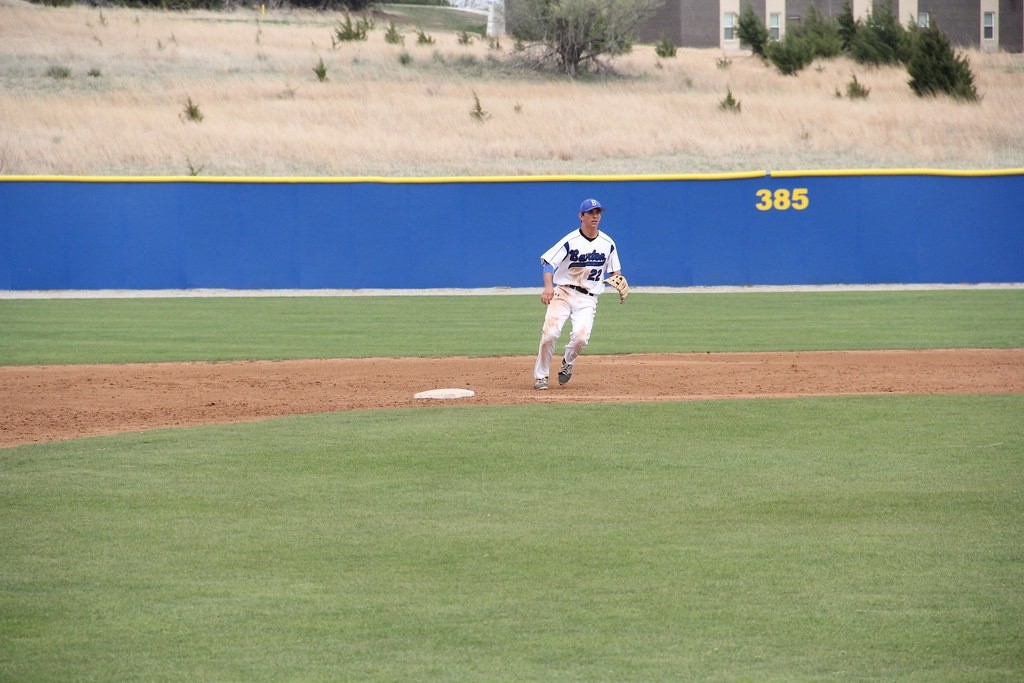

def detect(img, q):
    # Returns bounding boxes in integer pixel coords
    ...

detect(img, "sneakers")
[559,358,573,385]
[534,376,548,390]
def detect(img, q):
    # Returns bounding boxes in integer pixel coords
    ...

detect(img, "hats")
[579,197,605,213]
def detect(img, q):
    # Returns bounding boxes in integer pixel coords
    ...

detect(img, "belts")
[565,285,594,297]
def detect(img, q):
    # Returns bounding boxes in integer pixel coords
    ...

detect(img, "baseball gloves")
[601,275,630,304]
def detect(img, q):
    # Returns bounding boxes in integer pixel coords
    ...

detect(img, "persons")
[533,198,630,390]
